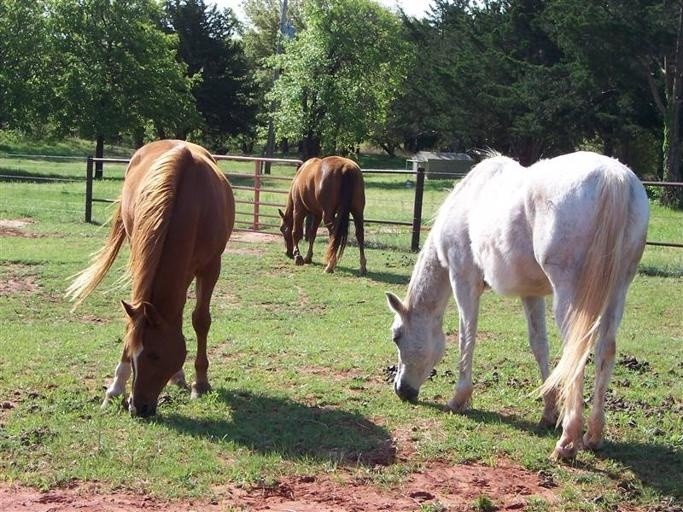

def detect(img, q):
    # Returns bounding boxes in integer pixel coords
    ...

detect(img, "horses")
[61,139,236,420]
[384,144,650,463]
[277,156,367,277]
